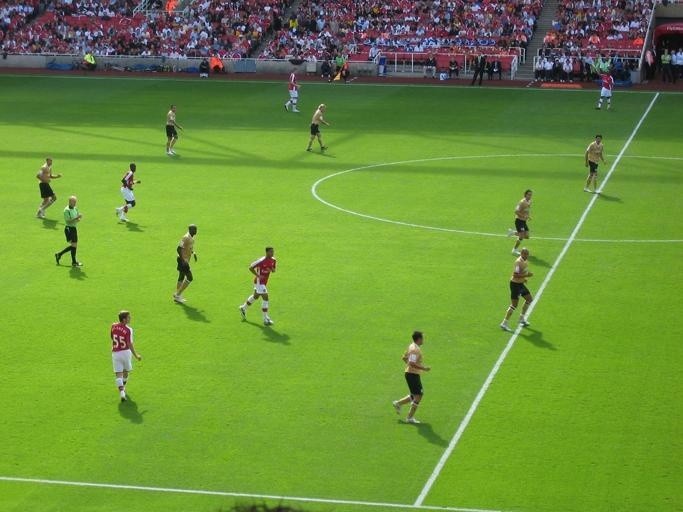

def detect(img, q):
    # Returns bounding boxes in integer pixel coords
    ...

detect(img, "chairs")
[0,0,657,85]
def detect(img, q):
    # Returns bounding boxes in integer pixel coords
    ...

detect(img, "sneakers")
[500,323,512,332]
[263,318,273,325]
[116,208,121,217]
[239,306,247,320]
[72,262,83,267]
[173,296,183,303]
[520,321,530,327]
[120,218,129,222]
[284,104,289,112]
[406,417,421,424]
[512,250,520,255]
[55,253,61,265]
[583,188,591,193]
[292,110,300,113]
[392,401,401,415]
[120,393,126,402]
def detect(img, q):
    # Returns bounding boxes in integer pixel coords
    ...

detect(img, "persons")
[285,68,302,112]
[35,158,62,218]
[55,196,83,266]
[165,105,184,156]
[306,104,330,151]
[597,73,615,109]
[115,163,141,224]
[506,189,533,256]
[584,135,607,193]
[3,0,683,85]
[392,331,431,424]
[238,247,276,325]
[500,248,534,332]
[173,224,198,302]
[110,311,141,401]
